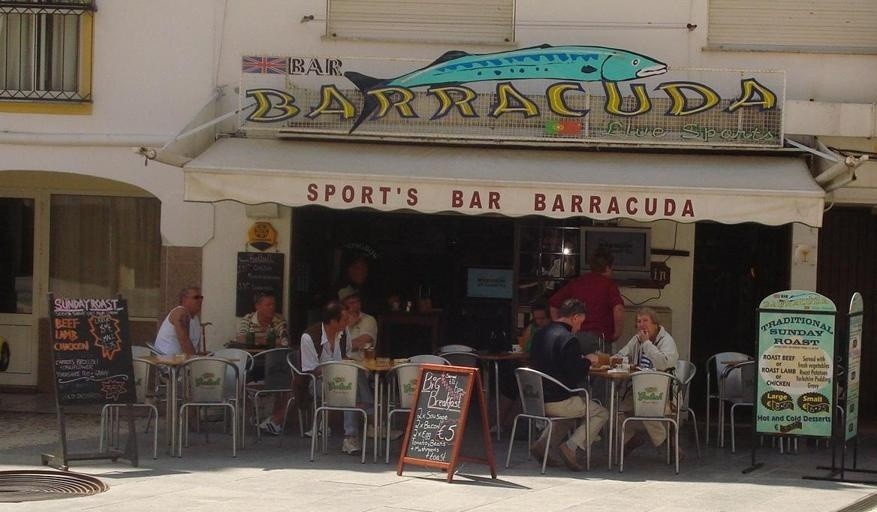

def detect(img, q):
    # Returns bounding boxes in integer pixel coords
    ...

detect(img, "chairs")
[706,352,756,447]
[278,352,322,454]
[97,354,161,460]
[144,338,195,449]
[206,348,254,401]
[312,360,374,463]
[406,355,452,365]
[720,361,796,454]
[506,366,599,474]
[386,363,420,464]
[667,361,702,465]
[610,366,683,477]
[433,342,510,442]
[178,357,240,458]
[242,347,301,444]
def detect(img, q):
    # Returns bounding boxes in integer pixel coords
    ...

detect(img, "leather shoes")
[367,423,403,439]
[533,439,555,465]
[556,442,582,474]
[670,447,686,463]
[623,435,646,454]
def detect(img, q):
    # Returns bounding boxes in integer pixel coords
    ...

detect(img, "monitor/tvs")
[579,226,650,280]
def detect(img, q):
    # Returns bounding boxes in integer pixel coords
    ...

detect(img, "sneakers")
[340,438,359,456]
[307,427,332,439]
[255,420,281,434]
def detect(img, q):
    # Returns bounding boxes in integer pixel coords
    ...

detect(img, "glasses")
[184,295,203,301]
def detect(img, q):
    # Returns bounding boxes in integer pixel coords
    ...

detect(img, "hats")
[337,285,359,299]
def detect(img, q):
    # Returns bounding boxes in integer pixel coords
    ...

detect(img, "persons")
[236,290,290,435]
[530,298,608,472]
[255,285,377,436]
[549,253,626,443]
[154,287,216,445]
[301,300,403,457]
[611,307,685,465]
[489,298,552,435]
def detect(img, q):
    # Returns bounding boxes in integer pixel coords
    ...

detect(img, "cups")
[377,357,390,367]
[512,344,524,357]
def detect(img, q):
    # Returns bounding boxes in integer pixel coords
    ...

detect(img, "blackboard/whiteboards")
[405,370,469,464]
[236,252,284,317]
[53,298,136,404]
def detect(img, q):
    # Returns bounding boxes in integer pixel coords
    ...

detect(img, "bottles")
[266,323,275,350]
[281,323,289,348]
[247,322,255,348]
